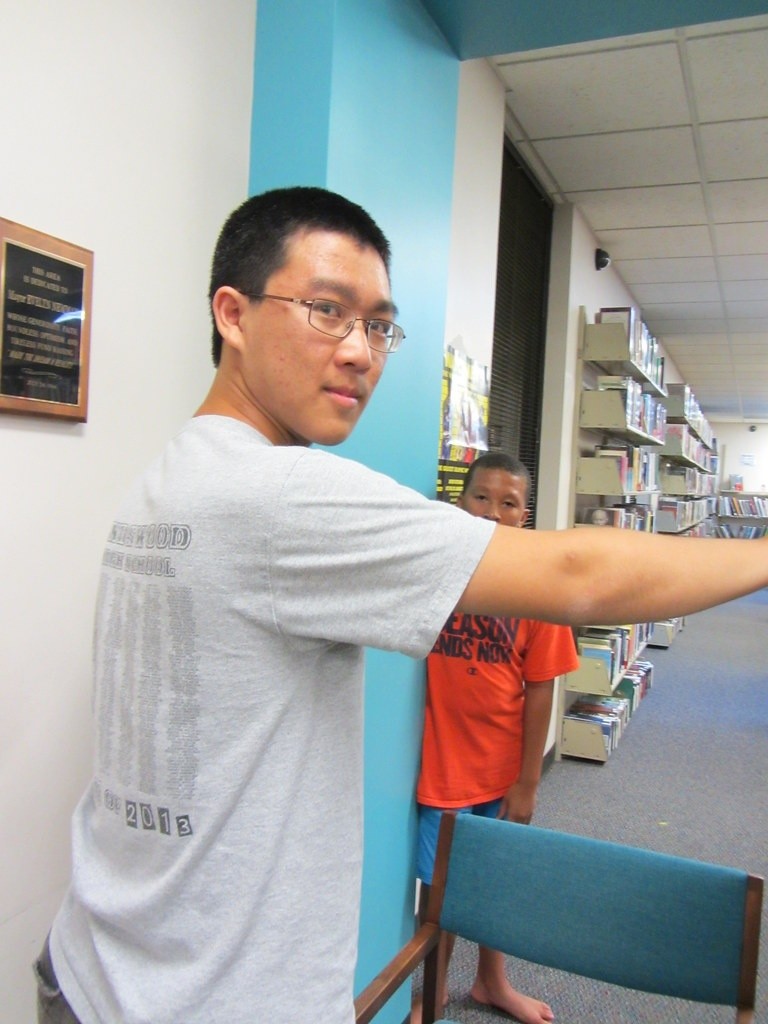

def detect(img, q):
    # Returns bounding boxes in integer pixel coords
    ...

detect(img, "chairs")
[353,809,763,1024]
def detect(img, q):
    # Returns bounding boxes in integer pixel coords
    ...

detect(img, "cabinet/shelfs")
[553,306,767,762]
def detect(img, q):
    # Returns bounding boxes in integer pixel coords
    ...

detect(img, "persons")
[442,396,454,460]
[32,188,767,1024]
[409,452,579,1024]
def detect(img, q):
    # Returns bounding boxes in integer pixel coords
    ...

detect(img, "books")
[565,305,768,757]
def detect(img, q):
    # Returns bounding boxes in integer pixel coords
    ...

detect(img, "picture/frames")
[0,216,94,424]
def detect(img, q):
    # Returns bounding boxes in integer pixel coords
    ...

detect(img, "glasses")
[238,289,406,354]
